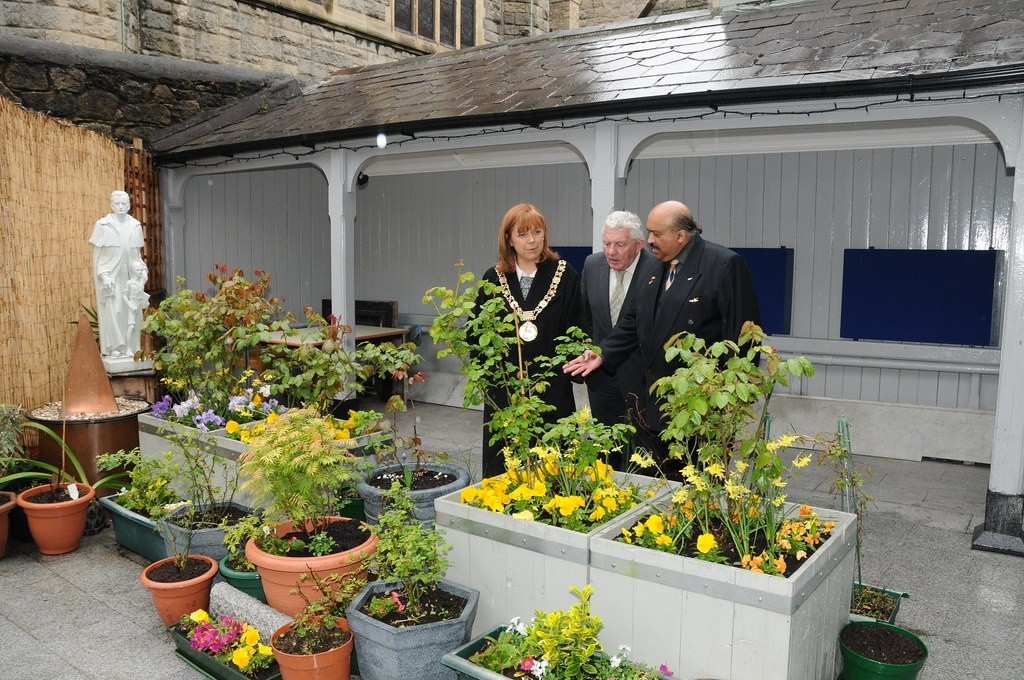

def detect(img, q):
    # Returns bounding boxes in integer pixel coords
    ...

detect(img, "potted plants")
[0,404,481,680]
[767,428,910,626]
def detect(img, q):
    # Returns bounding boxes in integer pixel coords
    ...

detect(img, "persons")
[465,204,580,481]
[562,200,760,491]
[89,191,148,356]
[123,261,150,357]
[578,211,663,478]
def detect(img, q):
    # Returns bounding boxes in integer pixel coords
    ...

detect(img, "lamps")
[356,171,369,185]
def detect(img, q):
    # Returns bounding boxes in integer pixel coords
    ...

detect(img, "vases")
[434,462,857,679]
[838,621,928,680]
[138,412,292,505]
[26,396,154,499]
[203,414,383,521]
[170,616,283,680]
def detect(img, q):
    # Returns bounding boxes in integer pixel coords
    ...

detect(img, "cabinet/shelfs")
[243,325,410,408]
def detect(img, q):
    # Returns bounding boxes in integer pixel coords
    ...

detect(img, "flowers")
[474,584,676,680]
[421,260,858,579]
[134,261,430,446]
[176,608,274,679]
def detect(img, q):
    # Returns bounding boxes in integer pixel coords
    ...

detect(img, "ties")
[664,258,680,292]
[610,270,625,328]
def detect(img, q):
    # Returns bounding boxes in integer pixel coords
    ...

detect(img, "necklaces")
[495,259,566,343]
